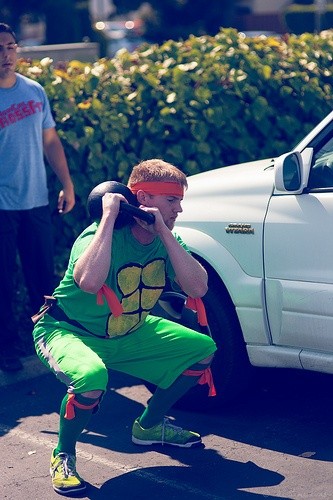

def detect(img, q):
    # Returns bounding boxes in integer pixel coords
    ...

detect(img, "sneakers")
[131,416,202,448]
[51,447,86,494]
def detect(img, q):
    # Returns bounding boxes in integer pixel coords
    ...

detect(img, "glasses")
[0,42,19,53]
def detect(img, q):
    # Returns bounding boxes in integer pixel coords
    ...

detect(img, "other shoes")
[0,351,24,371]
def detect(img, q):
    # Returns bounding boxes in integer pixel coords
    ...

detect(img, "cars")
[140,109,333,414]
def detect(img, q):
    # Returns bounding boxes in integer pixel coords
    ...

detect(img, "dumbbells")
[87,181,156,228]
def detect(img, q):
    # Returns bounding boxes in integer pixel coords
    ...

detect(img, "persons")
[0,23,74,372]
[31,159,218,493]
